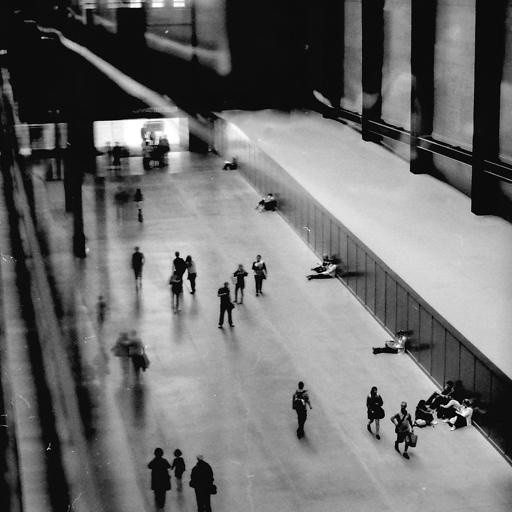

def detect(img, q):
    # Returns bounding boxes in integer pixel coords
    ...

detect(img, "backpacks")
[295,391,307,411]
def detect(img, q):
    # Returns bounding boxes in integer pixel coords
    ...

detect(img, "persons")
[222,158,238,170]
[104,137,170,223]
[254,193,275,213]
[366,387,385,440]
[372,330,408,355]
[95,245,340,378]
[390,401,413,459]
[147,447,172,512]
[171,449,185,491]
[189,454,217,512]
[292,381,312,440]
[411,380,474,431]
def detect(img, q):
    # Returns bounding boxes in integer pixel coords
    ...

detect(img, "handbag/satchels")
[405,433,418,448]
